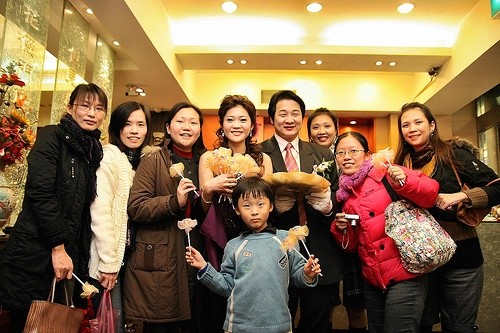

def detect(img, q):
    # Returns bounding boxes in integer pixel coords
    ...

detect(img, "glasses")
[70,100,107,115]
[335,149,365,158]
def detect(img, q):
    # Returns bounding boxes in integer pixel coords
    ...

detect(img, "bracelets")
[323,212,333,216]
[201,192,211,204]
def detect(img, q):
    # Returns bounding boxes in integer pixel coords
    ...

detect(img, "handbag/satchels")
[25,272,84,333]
[446,152,492,227]
[381,174,456,274]
[95,290,116,333]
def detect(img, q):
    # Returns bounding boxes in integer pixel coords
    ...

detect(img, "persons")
[330,132,440,333]
[120,102,208,333]
[198,94,274,333]
[395,103,500,333]
[307,107,369,333]
[87,102,154,333]
[255,90,340,333]
[185,176,321,333]
[0,83,108,333]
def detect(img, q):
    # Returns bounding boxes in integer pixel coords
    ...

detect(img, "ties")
[285,143,307,226]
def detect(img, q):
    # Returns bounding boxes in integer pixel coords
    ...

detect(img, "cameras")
[337,214,359,226]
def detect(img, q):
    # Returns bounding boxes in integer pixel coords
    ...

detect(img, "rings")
[437,202,440,205]
